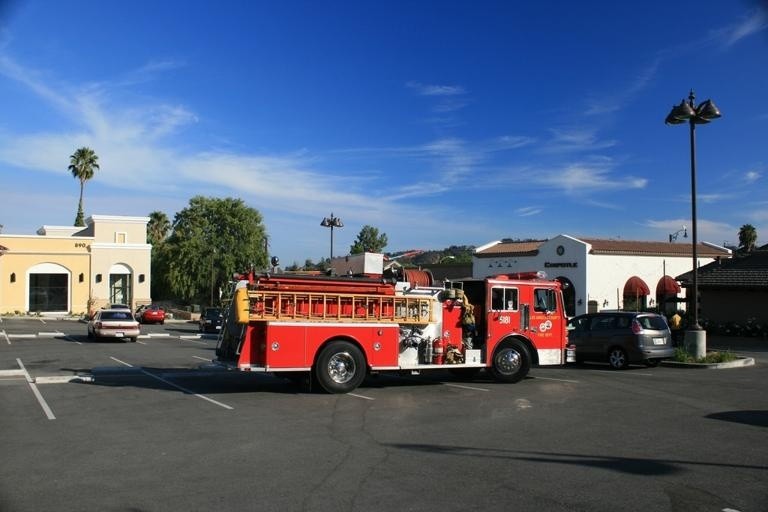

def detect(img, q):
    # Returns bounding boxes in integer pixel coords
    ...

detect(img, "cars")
[200,306,225,334]
[88,302,164,344]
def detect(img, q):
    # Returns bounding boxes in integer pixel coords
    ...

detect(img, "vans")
[567,309,674,371]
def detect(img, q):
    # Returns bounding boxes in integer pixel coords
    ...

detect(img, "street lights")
[663,87,723,331]
[320,211,345,259]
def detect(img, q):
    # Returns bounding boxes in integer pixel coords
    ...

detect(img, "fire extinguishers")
[422,335,443,364]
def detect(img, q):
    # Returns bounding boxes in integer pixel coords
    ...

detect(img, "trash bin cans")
[184,305,200,313]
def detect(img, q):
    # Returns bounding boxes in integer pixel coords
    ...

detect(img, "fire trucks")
[214,252,579,392]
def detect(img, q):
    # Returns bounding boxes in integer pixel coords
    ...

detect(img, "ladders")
[235,288,435,322]
